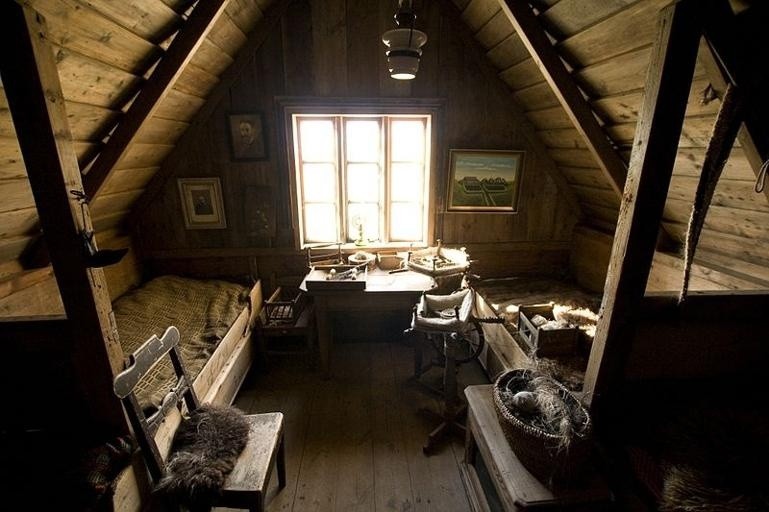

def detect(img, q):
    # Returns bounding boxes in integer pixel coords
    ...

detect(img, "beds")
[1,277,265,511]
[471,268,768,474]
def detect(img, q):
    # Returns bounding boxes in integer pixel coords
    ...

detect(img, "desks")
[298,265,437,378]
[462,382,613,510]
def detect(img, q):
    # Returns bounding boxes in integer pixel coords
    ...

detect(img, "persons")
[239,119,259,154]
[194,194,213,214]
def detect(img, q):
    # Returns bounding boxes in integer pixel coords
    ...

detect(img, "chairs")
[109,325,288,512]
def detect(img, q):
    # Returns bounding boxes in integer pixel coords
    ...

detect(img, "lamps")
[382,3,426,80]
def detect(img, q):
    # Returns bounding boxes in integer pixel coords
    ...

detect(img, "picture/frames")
[176,176,227,231]
[225,109,272,162]
[443,146,526,215]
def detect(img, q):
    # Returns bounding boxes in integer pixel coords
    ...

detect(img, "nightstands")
[253,298,317,374]
